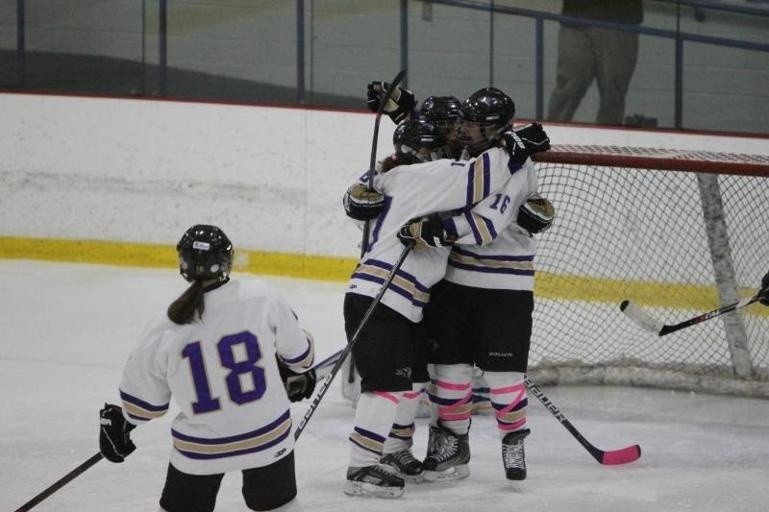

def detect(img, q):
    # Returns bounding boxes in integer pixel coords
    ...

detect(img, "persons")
[342,77,555,490]
[119,224,314,512]
[548,0,645,125]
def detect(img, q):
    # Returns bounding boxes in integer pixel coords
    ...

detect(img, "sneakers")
[348,417,530,486]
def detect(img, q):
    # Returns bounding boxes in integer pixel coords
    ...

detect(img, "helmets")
[177,225,234,281]
[393,88,515,163]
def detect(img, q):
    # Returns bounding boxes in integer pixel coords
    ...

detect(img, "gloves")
[398,217,455,249]
[518,197,554,233]
[368,81,418,124]
[275,352,316,401]
[99,402,136,462]
[344,182,385,219]
[500,122,552,172]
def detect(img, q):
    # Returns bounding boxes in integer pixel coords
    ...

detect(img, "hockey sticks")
[361,70,406,254]
[525,376,641,465]
[621,291,767,336]
